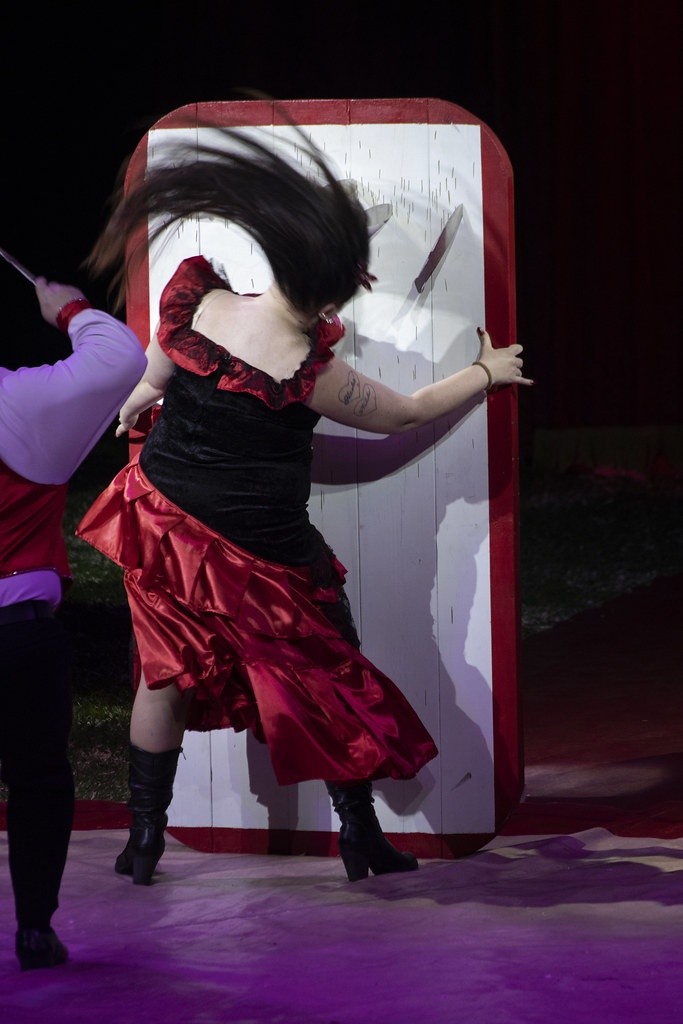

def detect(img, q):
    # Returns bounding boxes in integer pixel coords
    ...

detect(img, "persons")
[69,122,534,887]
[0,277,147,970]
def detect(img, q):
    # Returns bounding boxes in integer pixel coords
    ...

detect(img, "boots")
[325,775,418,883]
[115,742,178,887]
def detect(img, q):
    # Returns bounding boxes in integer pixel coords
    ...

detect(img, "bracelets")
[473,362,492,390]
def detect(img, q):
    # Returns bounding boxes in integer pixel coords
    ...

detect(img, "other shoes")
[14,928,69,971]
